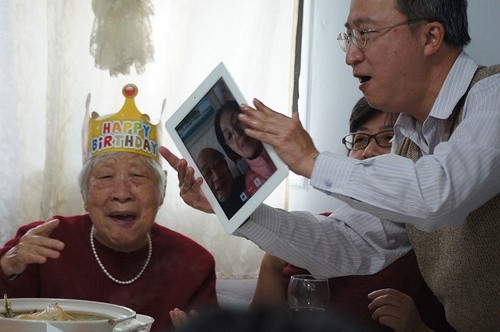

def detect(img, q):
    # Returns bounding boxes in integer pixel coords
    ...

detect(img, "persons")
[244,98,448,332]
[196,147,250,220]
[0,83,220,332]
[157,0,500,332]
[213,100,277,196]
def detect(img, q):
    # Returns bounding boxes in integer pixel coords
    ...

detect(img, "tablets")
[165,61,291,236]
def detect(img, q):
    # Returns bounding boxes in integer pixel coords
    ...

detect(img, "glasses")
[341,129,394,151]
[336,18,429,52]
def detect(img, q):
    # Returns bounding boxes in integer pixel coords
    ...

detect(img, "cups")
[287,273,330,325]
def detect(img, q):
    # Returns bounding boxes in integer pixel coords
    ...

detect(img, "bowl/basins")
[0,298,154,332]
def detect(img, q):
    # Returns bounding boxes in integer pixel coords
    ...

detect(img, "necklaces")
[91,224,152,284]
